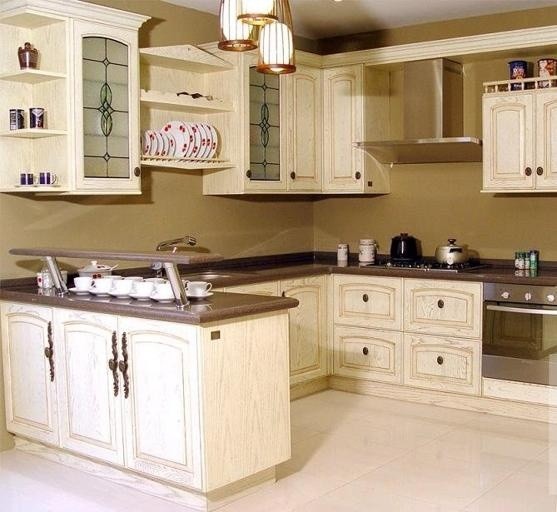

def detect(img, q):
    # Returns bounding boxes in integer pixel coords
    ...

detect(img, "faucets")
[156,235,197,251]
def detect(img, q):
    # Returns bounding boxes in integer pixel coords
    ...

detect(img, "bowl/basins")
[77,260,112,277]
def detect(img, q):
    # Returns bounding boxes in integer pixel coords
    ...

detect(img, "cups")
[36,272,52,289]
[39,172,56,184]
[19,172,34,185]
[73,275,212,295]
[53,270,68,286]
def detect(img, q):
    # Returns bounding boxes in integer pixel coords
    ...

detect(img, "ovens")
[481,282,557,387]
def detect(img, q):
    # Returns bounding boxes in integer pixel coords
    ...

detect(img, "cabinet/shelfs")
[480,87,557,194]
[53,308,203,490]
[224,275,330,386]
[285,64,321,195]
[71,18,142,196]
[201,52,287,196]
[323,63,366,195]
[329,271,484,401]
[0,5,70,196]
[138,43,233,170]
[0,301,53,445]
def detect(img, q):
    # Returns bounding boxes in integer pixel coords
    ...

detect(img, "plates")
[69,287,214,304]
[13,184,36,188]
[141,121,218,163]
[36,184,61,187]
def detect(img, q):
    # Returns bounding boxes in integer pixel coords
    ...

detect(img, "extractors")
[352,58,482,164]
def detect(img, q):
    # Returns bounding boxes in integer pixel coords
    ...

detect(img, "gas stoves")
[360,260,489,273]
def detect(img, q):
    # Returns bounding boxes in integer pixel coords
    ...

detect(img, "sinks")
[181,273,228,281]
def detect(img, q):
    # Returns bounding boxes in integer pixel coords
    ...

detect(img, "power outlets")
[219,0,296,74]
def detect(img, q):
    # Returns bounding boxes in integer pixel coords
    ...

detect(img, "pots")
[390,231,418,262]
[434,237,468,266]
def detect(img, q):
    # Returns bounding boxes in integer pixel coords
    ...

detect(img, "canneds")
[514,270,538,277]
[509,61,527,90]
[538,58,557,88]
[514,250,538,269]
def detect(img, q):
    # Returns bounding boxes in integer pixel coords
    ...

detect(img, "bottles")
[337,243,349,262]
[18,42,38,70]
[358,237,378,262]
[515,269,538,278]
[514,250,538,269]
